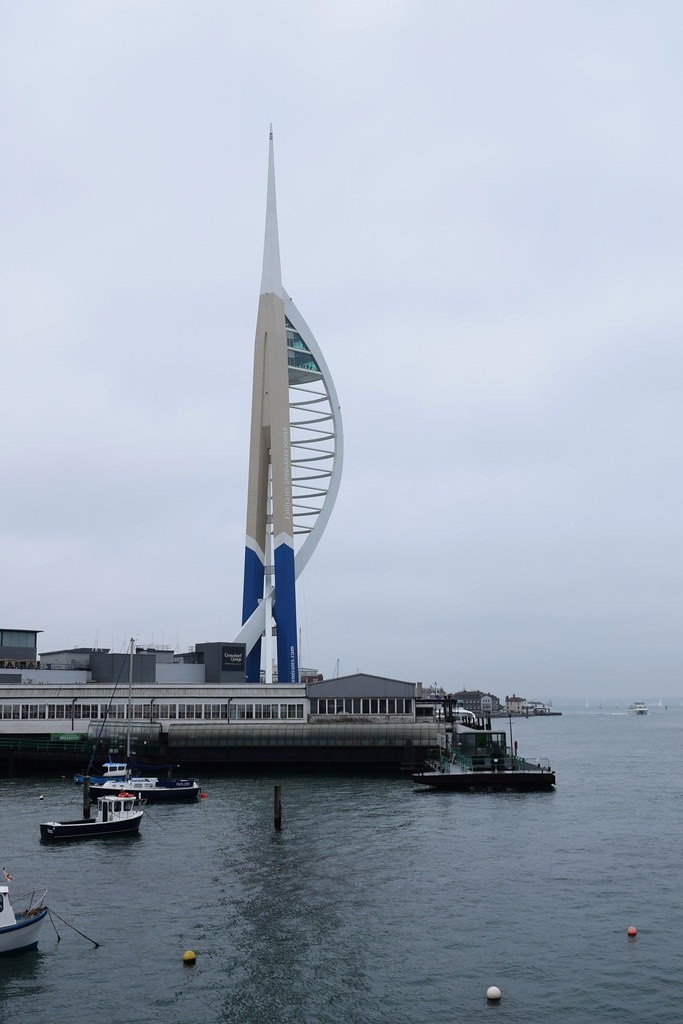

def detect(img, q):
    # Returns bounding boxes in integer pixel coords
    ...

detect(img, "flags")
[3,871,13,882]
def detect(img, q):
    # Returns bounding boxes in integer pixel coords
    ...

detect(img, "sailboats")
[72,638,202,801]
[583,697,665,709]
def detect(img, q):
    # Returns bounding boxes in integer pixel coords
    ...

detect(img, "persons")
[490,747,504,771]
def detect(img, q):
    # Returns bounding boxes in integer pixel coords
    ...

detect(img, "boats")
[0,874,52,952]
[628,701,649,716]
[410,696,555,791]
[39,789,148,843]
[534,707,562,716]
